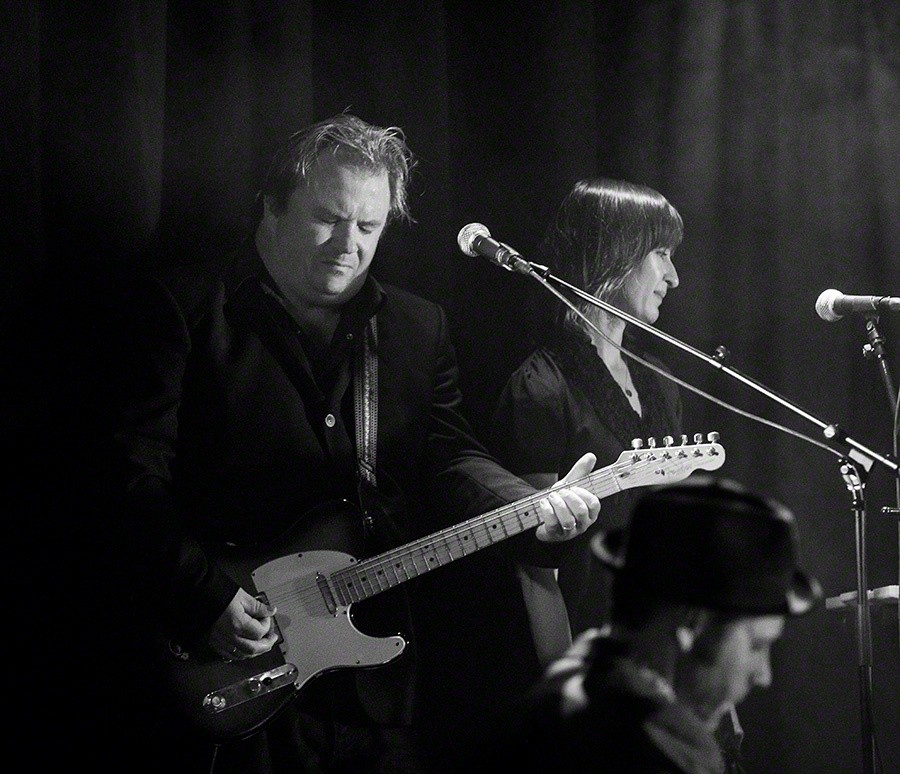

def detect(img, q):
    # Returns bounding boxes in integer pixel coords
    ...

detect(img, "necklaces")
[616,365,633,397]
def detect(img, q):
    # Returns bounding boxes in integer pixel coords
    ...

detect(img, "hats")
[623,479,815,617]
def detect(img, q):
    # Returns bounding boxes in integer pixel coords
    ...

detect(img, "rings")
[560,523,577,532]
[232,647,238,655]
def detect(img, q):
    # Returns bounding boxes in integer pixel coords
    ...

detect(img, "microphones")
[456,219,533,278]
[809,286,900,325]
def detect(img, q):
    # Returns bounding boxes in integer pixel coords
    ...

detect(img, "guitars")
[160,429,728,741]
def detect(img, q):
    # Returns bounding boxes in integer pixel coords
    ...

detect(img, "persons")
[115,113,823,774]
